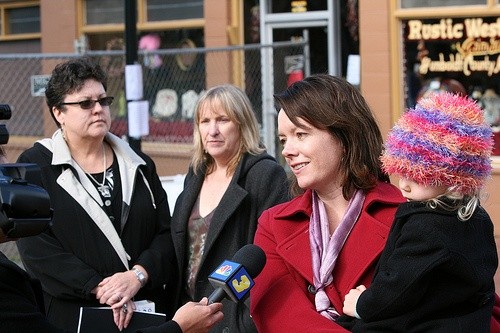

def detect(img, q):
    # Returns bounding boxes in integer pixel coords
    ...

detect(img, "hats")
[379,92,493,193]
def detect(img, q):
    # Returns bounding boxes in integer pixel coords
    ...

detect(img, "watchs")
[131,268,145,287]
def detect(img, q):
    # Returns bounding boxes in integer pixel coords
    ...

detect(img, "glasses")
[59,96,114,109]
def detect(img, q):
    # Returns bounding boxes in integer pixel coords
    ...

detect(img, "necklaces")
[69,144,110,196]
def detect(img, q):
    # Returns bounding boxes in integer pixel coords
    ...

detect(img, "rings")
[122,303,128,312]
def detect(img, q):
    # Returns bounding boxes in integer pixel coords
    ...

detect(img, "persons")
[171,84,288,333]
[249,74,409,333]
[16,58,171,333]
[0,144,44,333]
[334,91,499,333]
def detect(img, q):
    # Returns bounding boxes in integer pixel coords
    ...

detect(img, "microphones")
[205,245,266,306]
